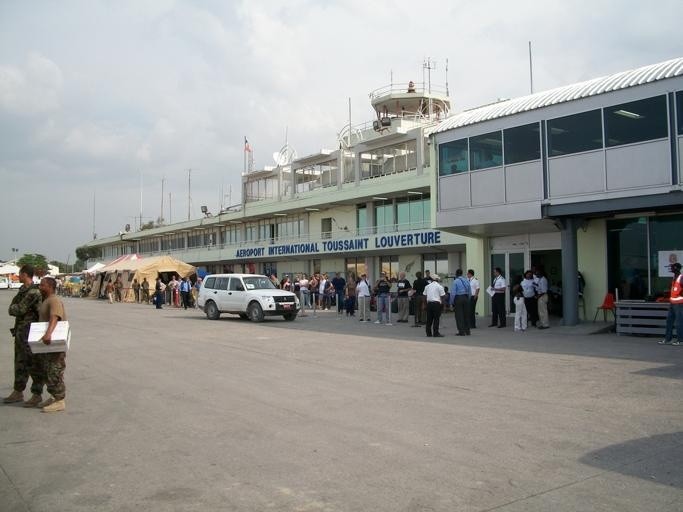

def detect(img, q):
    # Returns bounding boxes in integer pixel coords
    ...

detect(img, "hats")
[430,273,440,281]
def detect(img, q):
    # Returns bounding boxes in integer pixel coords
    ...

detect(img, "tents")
[80,254,197,302]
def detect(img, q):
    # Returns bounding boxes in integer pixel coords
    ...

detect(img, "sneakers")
[658,339,673,345]
[495,325,506,329]
[671,340,682,346]
[4,390,24,404]
[426,330,471,337]
[359,317,408,325]
[24,393,42,408]
[40,396,64,413]
[488,323,497,327]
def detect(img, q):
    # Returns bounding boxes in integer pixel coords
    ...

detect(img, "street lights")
[11,248,19,265]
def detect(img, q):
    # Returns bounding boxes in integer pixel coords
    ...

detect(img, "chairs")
[593,293,615,323]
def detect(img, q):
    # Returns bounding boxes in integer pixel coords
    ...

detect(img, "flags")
[245,137,256,152]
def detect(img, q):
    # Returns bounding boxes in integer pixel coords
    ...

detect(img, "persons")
[270,269,480,337]
[657,263,683,345]
[39,277,66,412]
[512,269,549,332]
[5,265,43,407]
[55,277,203,310]
[488,268,507,328]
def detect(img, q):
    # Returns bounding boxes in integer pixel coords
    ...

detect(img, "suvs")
[196,272,301,324]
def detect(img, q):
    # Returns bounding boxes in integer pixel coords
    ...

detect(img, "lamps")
[201,206,211,218]
